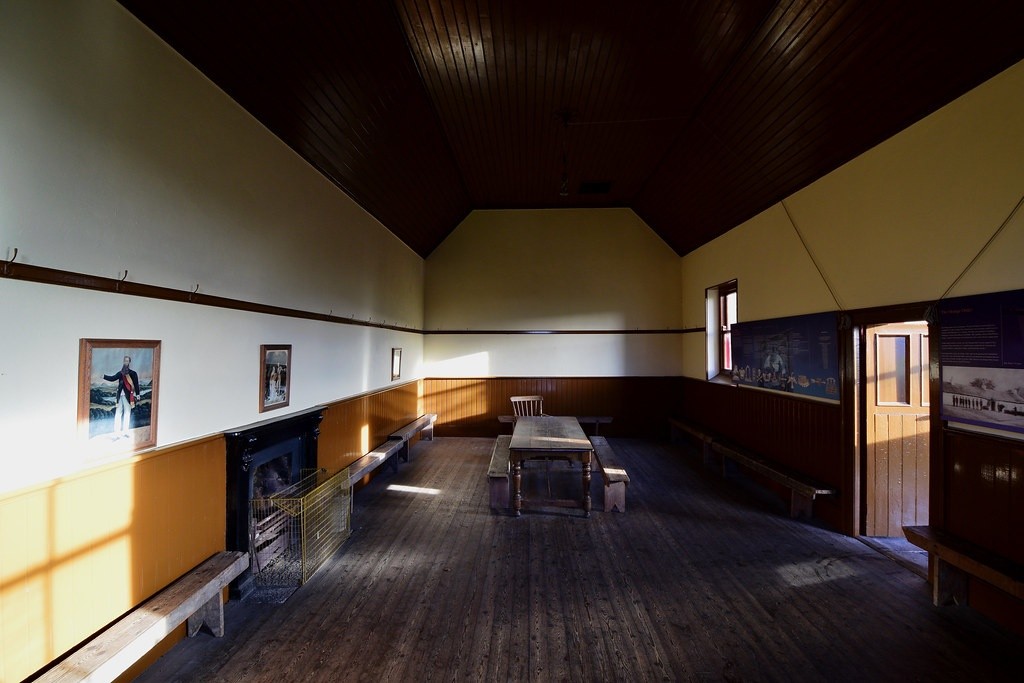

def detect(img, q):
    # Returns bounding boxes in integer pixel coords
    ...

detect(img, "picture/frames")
[259,344,292,412]
[392,347,402,380]
[77,338,162,462]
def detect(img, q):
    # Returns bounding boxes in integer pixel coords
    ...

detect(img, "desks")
[509,416,594,519]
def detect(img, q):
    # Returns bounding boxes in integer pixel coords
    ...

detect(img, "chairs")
[510,396,549,417]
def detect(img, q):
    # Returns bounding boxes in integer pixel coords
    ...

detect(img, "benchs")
[497,415,613,436]
[487,435,511,509]
[670,409,835,520]
[590,436,630,513]
[30,550,251,683]
[390,414,438,463]
[901,525,1024,607]
[339,439,403,515]
